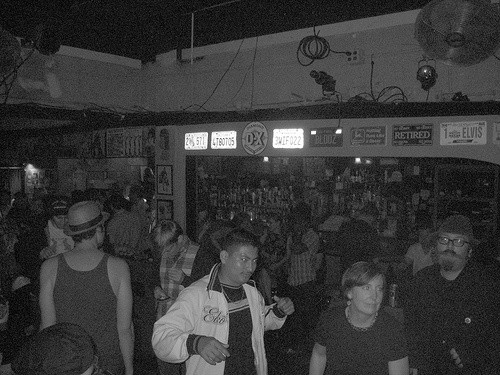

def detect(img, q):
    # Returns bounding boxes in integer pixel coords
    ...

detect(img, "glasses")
[436,236,472,247]
[159,244,174,253]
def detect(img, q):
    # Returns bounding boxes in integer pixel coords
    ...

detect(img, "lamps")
[417,59,436,90]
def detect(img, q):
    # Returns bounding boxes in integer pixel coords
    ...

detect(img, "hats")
[426,214,481,247]
[52,197,70,215]
[63,200,111,236]
[291,201,312,217]
[413,210,435,228]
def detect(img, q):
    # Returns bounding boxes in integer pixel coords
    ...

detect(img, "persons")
[0,189,499,375]
[39,201,135,375]
[151,229,294,375]
[309,261,410,375]
